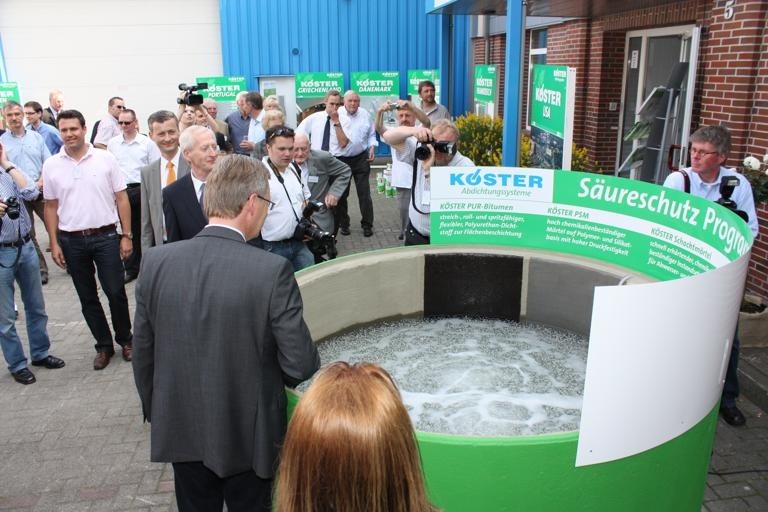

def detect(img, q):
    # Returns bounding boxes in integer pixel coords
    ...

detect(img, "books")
[623,121,653,141]
[635,85,666,116]
[617,145,646,173]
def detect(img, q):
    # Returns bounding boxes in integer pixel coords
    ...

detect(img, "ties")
[322,117,330,151]
[166,161,175,186]
[199,183,204,218]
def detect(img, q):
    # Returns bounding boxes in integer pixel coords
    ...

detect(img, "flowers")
[572,141,602,174]
[452,114,532,167]
[742,153,768,210]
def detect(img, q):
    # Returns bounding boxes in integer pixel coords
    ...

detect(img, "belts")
[127,184,139,189]
[1,235,31,248]
[70,225,115,237]
[271,237,296,245]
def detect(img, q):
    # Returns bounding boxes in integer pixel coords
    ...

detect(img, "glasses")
[118,120,135,125]
[268,128,295,140]
[688,147,717,157]
[117,106,126,109]
[248,195,277,212]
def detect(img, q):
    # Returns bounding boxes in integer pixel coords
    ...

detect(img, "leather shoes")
[12,367,35,383]
[364,228,373,237]
[94,351,114,370]
[341,226,350,235]
[121,342,133,363]
[39,271,49,284]
[718,405,746,426]
[124,273,138,284]
[32,356,65,368]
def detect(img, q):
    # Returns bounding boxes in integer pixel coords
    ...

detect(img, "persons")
[128,152,323,512]
[661,122,761,428]
[1,81,479,387]
[266,360,441,512]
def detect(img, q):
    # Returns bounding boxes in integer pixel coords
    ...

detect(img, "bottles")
[375,170,398,199]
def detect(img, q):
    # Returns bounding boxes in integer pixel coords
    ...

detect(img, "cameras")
[2,196,21,219]
[414,134,457,160]
[714,176,749,223]
[390,103,399,110]
[295,199,330,245]
